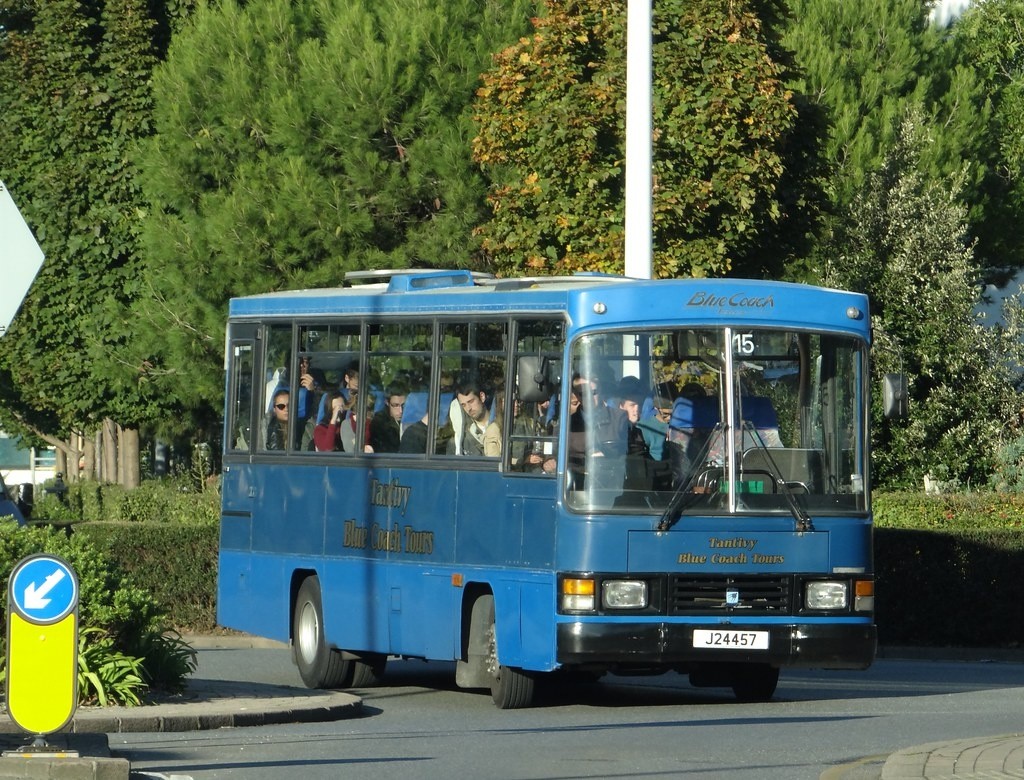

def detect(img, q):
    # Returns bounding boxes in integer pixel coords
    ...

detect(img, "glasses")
[390,401,404,409]
[275,402,289,410]
[577,390,597,396]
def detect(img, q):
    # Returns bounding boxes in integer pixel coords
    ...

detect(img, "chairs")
[243,352,790,508]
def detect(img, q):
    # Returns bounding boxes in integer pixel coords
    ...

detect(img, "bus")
[211,260,911,714]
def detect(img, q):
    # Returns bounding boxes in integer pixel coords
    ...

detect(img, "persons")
[263,347,670,473]
